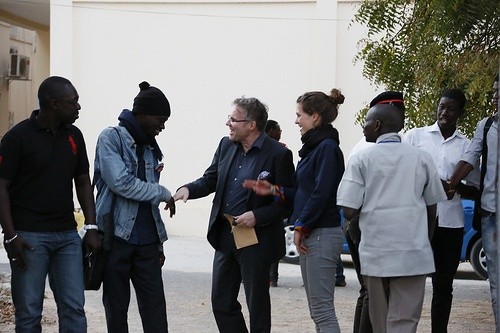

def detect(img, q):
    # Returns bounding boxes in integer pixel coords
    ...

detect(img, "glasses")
[227,114,250,123]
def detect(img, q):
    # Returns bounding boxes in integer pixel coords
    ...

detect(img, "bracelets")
[84,224,98,230]
[4,233,18,243]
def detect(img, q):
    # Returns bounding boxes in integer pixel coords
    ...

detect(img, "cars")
[282,185,489,281]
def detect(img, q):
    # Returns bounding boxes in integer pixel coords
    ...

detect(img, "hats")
[370,90,407,110]
[131,81,171,116]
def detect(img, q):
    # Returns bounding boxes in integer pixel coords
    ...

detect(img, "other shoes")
[269,272,278,288]
[334,274,347,287]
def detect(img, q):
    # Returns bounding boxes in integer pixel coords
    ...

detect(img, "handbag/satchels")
[81,225,107,290]
[472,191,484,231]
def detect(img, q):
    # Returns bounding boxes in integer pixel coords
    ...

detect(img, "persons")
[266,120,281,287]
[296,88,345,333]
[401,90,480,333]
[342,91,452,333]
[173,98,294,333]
[337,106,448,333]
[335,255,346,287]
[96,81,175,333]
[447,76,500,333]
[0,76,100,333]
